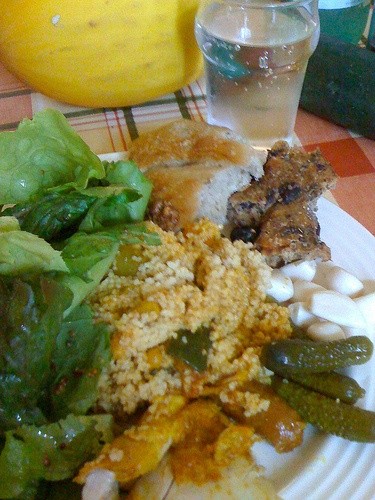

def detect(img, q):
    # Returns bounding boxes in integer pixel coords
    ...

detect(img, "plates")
[99,151,372,500]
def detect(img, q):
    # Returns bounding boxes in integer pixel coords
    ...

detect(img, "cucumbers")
[286,370,361,404]
[263,335,373,375]
[275,380,375,443]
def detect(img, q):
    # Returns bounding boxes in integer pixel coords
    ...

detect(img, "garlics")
[263,258,375,342]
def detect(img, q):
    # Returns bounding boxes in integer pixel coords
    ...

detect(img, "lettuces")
[0,109,154,500]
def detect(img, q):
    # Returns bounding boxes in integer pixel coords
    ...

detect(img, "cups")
[194,0,320,161]
